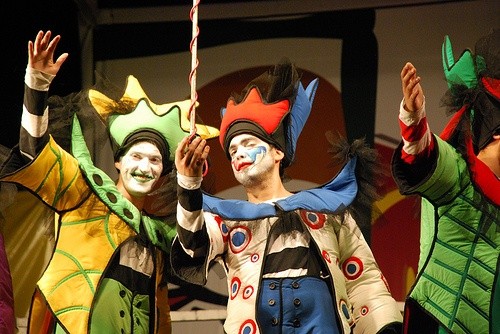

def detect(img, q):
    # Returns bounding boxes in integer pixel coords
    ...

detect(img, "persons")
[167,84,405,334]
[386,62,499,334]
[1,30,180,334]
